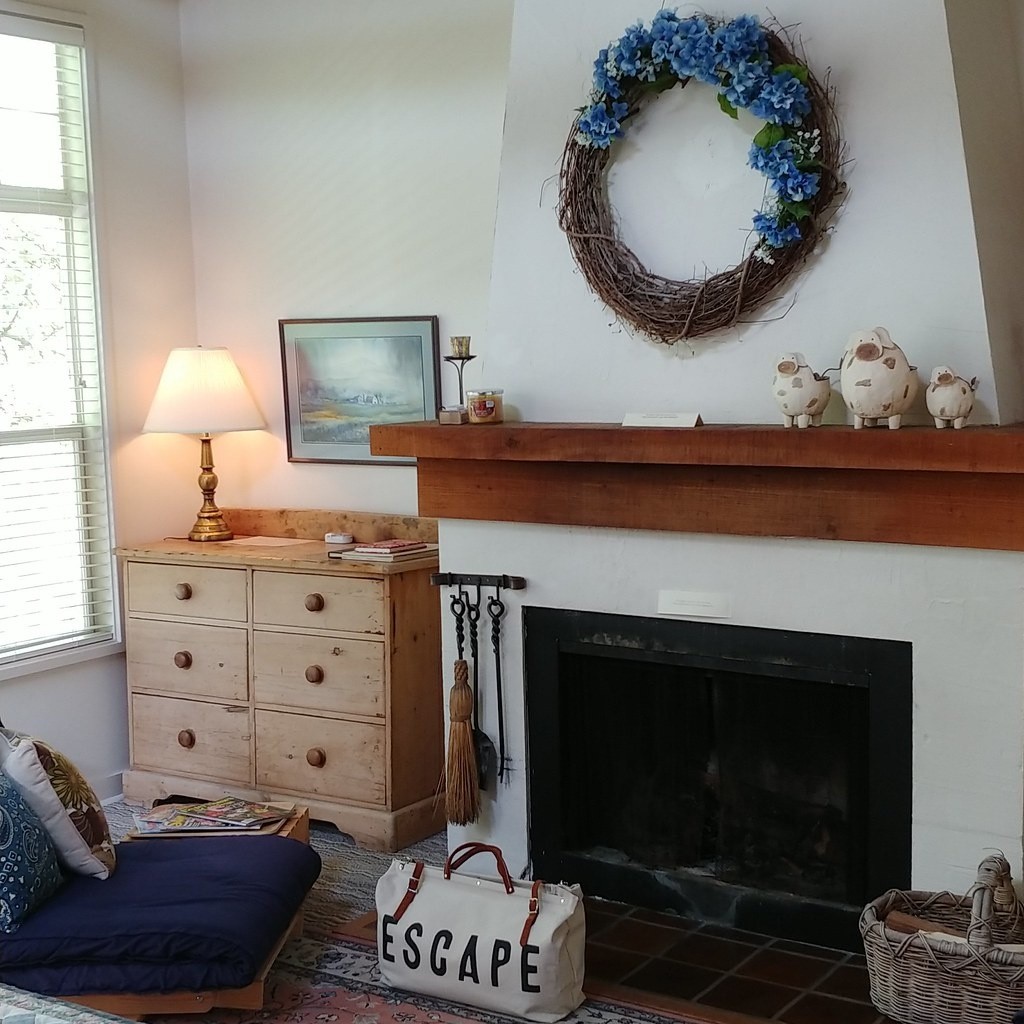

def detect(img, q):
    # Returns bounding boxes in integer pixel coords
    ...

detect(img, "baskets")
[859,855,1023,1024]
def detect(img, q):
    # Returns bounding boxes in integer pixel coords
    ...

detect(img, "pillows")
[0,727,118,882]
[1,772,74,934]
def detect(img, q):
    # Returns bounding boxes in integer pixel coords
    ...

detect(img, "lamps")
[143,346,266,544]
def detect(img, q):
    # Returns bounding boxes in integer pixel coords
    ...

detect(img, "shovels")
[467,603,495,792]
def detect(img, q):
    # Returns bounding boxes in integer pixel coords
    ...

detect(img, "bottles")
[467,388,505,425]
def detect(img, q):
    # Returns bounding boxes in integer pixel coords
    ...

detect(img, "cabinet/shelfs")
[116,507,454,852]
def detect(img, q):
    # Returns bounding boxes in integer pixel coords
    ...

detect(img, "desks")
[120,803,311,846]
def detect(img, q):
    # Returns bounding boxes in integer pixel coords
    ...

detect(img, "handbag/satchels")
[376,841,588,1022]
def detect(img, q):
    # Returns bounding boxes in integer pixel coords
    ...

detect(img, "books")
[131,796,298,834]
[327,539,438,563]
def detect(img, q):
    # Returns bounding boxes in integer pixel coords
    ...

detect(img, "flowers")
[577,3,836,266]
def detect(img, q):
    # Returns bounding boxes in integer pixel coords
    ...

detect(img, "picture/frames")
[277,316,446,467]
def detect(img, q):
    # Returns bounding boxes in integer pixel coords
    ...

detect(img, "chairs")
[0,720,321,1017]
